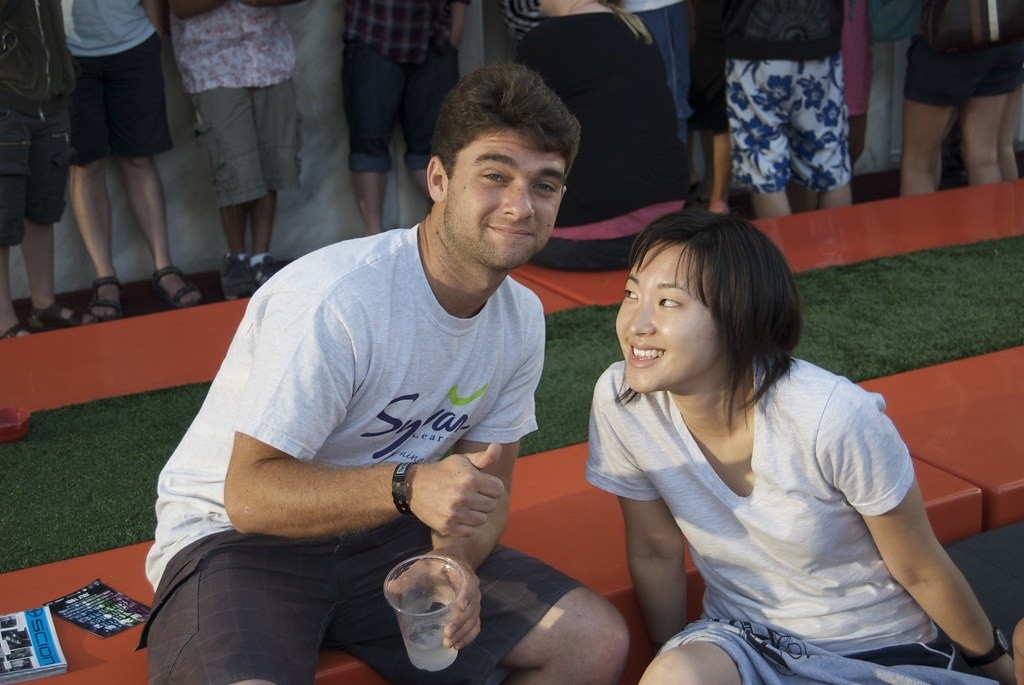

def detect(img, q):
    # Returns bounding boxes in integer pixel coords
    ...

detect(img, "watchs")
[958,627,1009,668]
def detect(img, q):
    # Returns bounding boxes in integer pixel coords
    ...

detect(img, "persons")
[136,62,629,685]
[0,0,1024,339]
[585,209,1024,685]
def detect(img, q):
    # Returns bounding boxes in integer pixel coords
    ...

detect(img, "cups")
[384,555,465,671]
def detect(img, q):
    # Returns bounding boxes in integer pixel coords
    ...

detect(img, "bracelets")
[392,461,419,514]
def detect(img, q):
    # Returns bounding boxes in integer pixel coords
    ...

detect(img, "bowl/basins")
[0,406,30,441]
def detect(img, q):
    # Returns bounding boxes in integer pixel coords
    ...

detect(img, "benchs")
[0,345,1024,683]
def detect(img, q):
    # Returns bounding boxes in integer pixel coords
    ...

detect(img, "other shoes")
[221,252,256,299]
[252,251,282,287]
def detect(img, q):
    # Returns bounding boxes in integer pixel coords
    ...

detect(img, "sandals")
[26,301,98,329]
[87,277,123,322]
[152,266,204,307]
[0,324,24,338]
